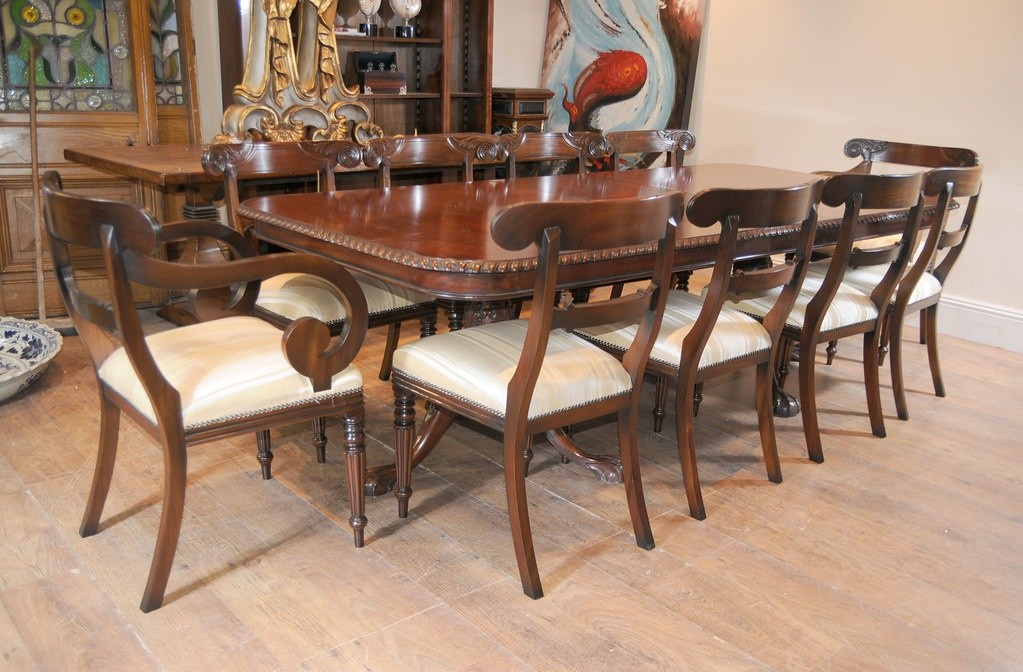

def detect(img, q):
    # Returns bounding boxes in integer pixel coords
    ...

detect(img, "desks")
[64,130,608,327]
[239,163,962,494]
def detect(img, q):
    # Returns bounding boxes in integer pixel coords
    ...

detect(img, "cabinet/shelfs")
[334,0,494,134]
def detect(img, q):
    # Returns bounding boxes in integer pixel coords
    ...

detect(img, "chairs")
[42,126,984,614]
